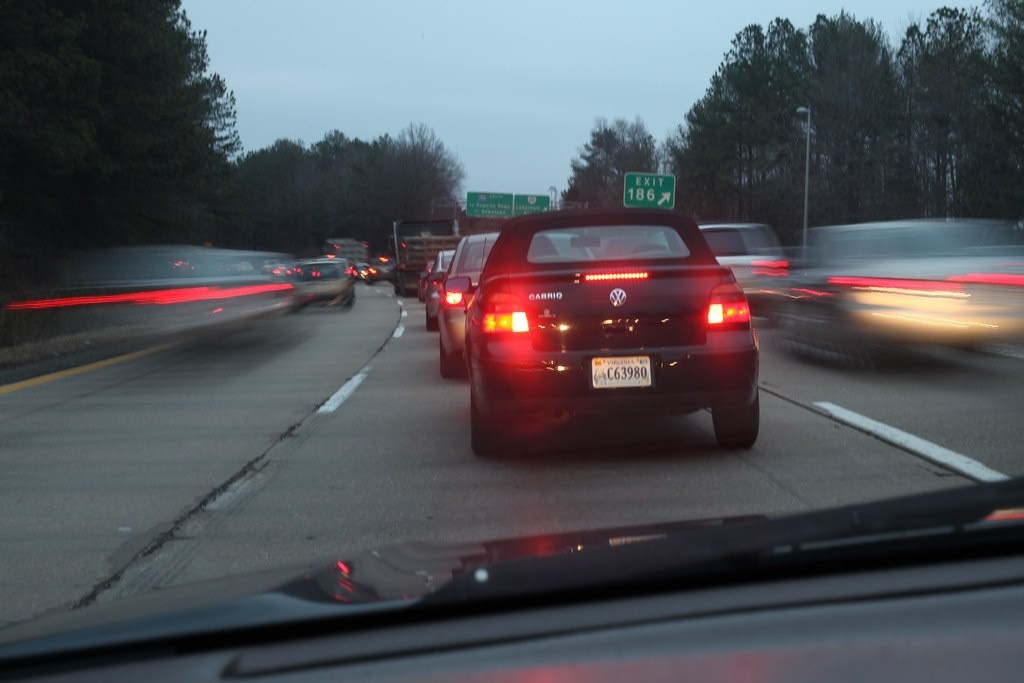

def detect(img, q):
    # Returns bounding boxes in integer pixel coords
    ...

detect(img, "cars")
[655,222,792,327]
[425,230,598,378]
[291,251,397,311]
[777,219,1024,369]
[416,247,457,331]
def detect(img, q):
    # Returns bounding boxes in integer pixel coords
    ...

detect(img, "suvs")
[441,208,763,459]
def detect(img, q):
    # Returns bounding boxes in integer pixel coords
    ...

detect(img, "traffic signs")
[513,194,550,217]
[623,173,676,210]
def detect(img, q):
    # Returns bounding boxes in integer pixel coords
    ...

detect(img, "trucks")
[389,218,453,299]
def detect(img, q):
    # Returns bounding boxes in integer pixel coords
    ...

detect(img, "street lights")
[796,105,812,272]
